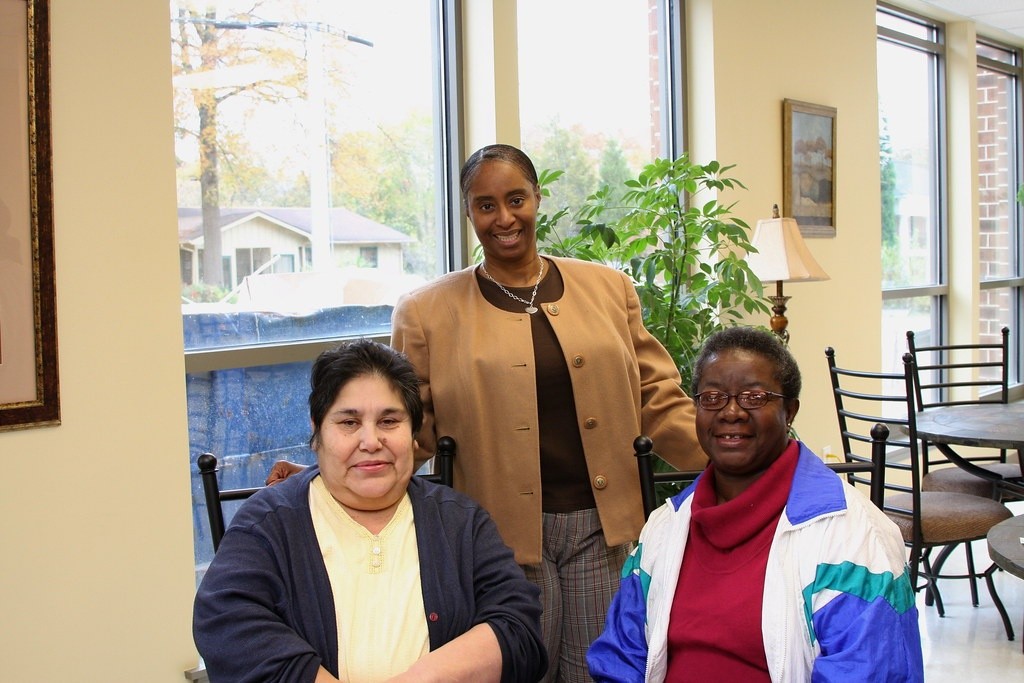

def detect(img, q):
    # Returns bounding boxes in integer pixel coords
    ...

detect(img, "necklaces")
[482,256,544,314]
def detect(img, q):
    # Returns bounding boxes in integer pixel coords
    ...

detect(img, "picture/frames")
[783,97,838,240]
[0,1,66,431]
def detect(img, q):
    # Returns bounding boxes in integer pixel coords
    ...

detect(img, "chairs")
[198,434,457,561]
[633,327,1023,641]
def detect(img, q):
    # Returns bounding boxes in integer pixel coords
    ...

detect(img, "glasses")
[694,390,792,411]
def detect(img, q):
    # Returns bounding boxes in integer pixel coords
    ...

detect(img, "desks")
[898,404,1024,605]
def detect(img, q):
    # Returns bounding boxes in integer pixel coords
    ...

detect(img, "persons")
[266,143,712,683]
[192,339,548,683]
[585,327,924,683]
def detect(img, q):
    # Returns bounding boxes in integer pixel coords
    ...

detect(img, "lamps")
[742,206,828,348]
